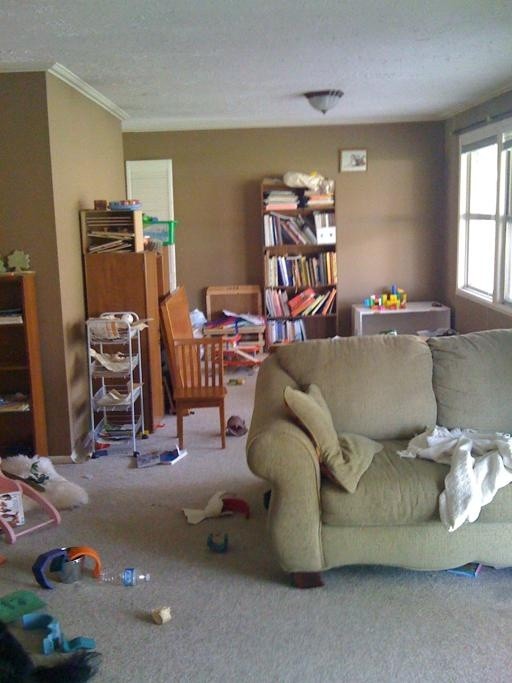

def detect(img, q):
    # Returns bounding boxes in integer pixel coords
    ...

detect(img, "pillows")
[284,383,382,493]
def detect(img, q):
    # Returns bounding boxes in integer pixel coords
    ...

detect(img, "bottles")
[98,566,150,587]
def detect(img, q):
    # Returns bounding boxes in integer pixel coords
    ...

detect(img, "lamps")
[302,87,344,117]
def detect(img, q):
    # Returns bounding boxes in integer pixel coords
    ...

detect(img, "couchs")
[244,328,512,589]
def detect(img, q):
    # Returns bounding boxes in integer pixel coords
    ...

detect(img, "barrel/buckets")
[56,546,85,584]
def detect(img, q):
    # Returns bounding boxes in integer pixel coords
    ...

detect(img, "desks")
[351,301,450,336]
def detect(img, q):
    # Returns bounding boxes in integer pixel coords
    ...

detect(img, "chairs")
[171,338,227,451]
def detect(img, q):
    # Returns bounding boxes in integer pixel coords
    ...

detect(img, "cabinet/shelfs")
[1,271,47,459]
[260,183,339,346]
[206,290,262,358]
[83,211,170,458]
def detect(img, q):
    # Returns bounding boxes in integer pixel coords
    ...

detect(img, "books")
[0,312,23,324]
[447,563,482,577]
[159,443,189,465]
[0,491,25,530]
[264,189,337,343]
[0,390,31,412]
[137,447,161,468]
